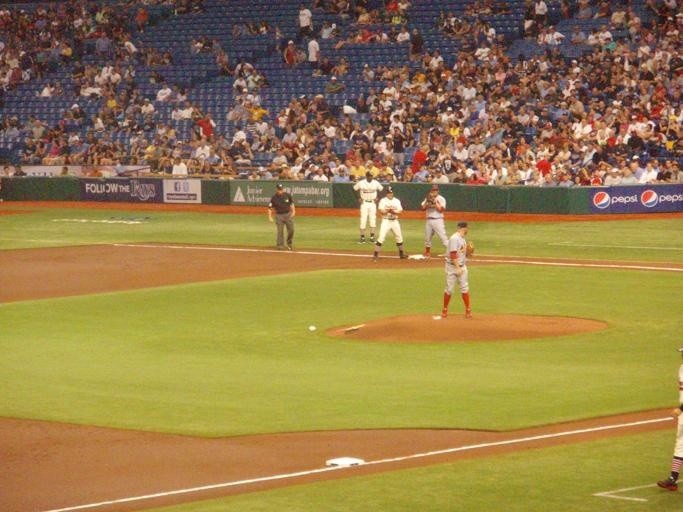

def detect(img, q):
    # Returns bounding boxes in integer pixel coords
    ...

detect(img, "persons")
[419,184,449,257]
[370,184,409,260]
[1,2,683,188]
[656,347,683,493]
[264,182,297,250]
[438,221,476,320]
[351,170,383,244]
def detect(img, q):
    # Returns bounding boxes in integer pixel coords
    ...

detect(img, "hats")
[365,171,373,177]
[432,184,439,191]
[386,186,393,193]
[457,221,468,228]
[276,184,283,191]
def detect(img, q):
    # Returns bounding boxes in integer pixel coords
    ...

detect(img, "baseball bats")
[345,323,365,333]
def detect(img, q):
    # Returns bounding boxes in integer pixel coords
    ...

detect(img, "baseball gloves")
[467,243,473,257]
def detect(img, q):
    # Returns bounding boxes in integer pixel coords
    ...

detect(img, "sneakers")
[277,234,431,259]
[441,307,448,320]
[465,307,473,319]
[657,476,677,491]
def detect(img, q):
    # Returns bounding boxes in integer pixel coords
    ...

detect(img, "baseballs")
[308,326,316,331]
